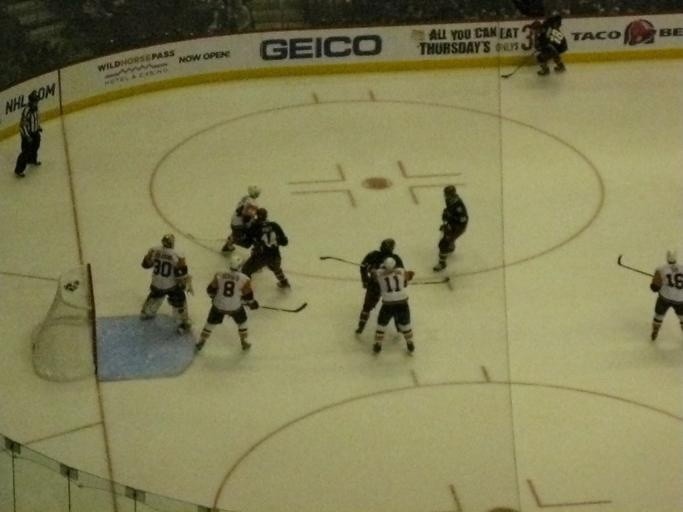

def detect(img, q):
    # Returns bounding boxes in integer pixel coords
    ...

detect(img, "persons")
[222,184,262,253]
[650,250,683,340]
[433,185,468,271]
[236,208,290,287]
[195,255,259,353]
[15,93,43,177]
[290,1,683,29]
[366,257,415,353]
[356,239,405,334]
[0,0,254,92]
[141,233,194,336]
[530,15,568,76]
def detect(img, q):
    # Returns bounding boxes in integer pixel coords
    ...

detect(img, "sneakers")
[19,173,25,176]
[407,343,415,351]
[277,279,288,286]
[374,345,380,352]
[652,332,658,339]
[355,328,362,333]
[195,341,202,350]
[240,343,250,349]
[433,262,446,270]
[537,68,549,74]
[221,244,235,250]
[554,64,565,71]
[36,162,42,165]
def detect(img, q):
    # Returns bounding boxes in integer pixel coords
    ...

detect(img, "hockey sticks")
[501,50,536,78]
[242,302,307,313]
[406,276,451,284]
[618,255,654,277]
[320,256,369,269]
[187,233,227,241]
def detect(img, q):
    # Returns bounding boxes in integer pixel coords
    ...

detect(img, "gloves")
[361,262,371,270]
[248,301,258,309]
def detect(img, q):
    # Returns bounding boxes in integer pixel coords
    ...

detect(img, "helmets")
[531,21,541,30]
[229,257,242,269]
[248,184,261,197]
[445,186,456,196]
[666,250,677,261]
[257,208,267,219]
[162,234,174,247]
[381,238,395,251]
[384,257,396,269]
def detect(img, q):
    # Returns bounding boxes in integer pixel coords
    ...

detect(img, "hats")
[28,94,40,103]
